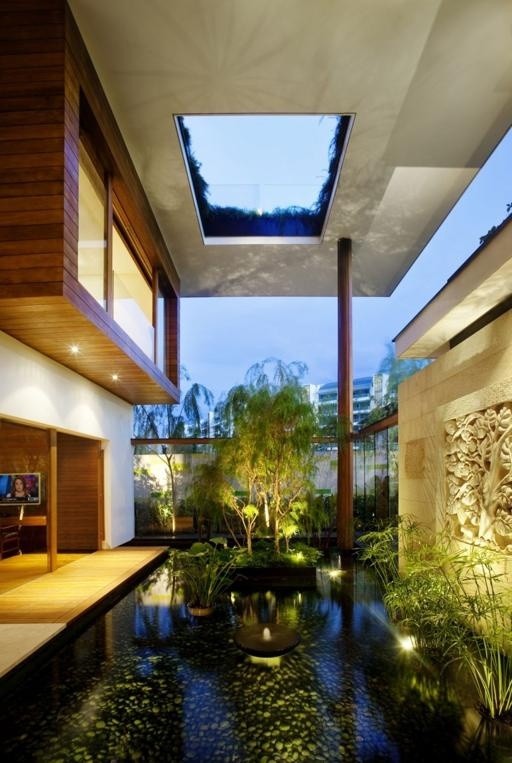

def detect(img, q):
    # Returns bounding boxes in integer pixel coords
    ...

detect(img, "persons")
[6,477,33,498]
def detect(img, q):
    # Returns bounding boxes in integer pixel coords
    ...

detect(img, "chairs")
[0,523,21,557]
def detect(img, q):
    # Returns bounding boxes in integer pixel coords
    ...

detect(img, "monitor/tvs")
[0,472,42,506]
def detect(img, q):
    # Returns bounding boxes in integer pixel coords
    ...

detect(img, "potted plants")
[165,536,241,617]
